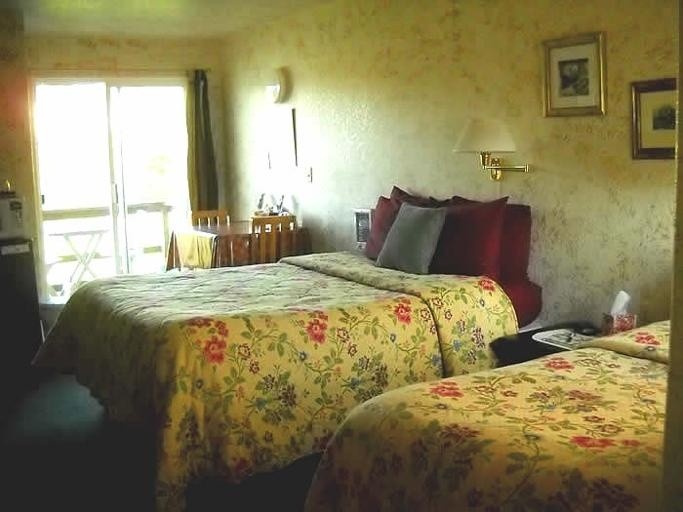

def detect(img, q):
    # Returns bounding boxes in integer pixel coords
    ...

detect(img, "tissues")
[602,289,638,336]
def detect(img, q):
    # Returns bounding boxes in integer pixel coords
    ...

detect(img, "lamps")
[452,117,530,179]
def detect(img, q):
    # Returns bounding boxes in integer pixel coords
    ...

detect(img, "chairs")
[252,215,298,263]
[191,210,224,226]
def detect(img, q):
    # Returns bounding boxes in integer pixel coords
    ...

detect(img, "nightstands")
[491,322,602,366]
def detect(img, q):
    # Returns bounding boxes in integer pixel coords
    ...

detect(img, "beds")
[28,251,542,511]
[305,320,672,510]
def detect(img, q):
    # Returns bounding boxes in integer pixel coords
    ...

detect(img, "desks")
[46,227,110,284]
[166,220,306,272]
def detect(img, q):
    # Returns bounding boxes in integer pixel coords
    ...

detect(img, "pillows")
[367,196,438,274]
[376,200,448,275]
[390,185,450,224]
[449,196,531,283]
[427,196,508,275]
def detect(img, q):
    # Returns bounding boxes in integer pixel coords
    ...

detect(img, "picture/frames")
[541,30,608,116]
[629,78,676,159]
